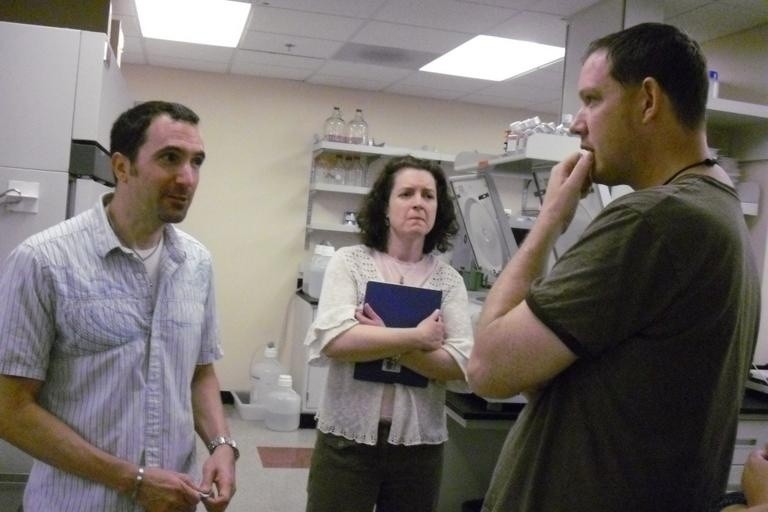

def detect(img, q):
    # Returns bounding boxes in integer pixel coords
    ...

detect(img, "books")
[352,279,443,387]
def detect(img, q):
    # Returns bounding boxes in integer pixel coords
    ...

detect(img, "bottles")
[343,208,358,225]
[325,106,347,142]
[303,238,335,297]
[249,343,289,405]
[314,153,377,187]
[708,70,719,97]
[348,109,369,146]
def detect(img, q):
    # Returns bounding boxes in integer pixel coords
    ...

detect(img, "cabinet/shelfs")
[305,140,456,252]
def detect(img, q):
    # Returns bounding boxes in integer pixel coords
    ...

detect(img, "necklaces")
[382,251,425,287]
[106,203,164,262]
[659,153,724,187]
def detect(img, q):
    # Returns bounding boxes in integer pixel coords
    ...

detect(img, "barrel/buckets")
[308,245,335,297]
[301,244,325,296]
[247,340,285,405]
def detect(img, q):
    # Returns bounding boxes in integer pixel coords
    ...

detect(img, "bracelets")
[130,462,145,500]
[207,437,240,462]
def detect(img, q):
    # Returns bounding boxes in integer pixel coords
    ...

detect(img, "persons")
[1,98,240,511]
[463,20,762,511]
[741,446,768,511]
[302,154,475,510]
